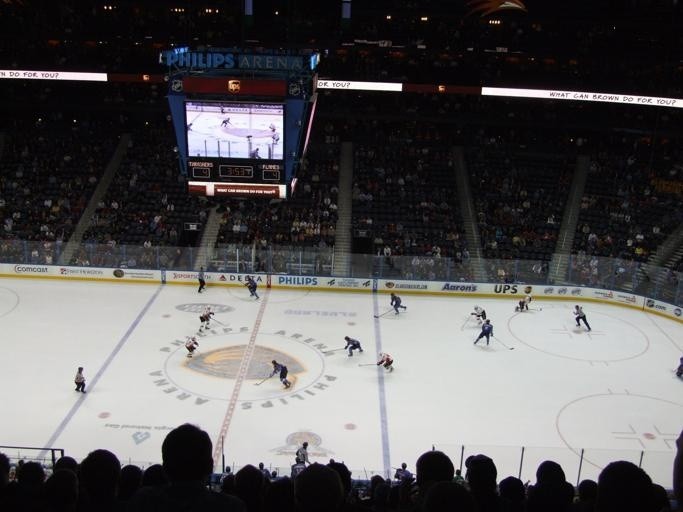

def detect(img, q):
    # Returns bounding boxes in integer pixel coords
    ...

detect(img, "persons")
[74,367,86,393]
[573,305,591,331]
[1,420,683,511]
[474,319,494,345]
[675,357,683,377]
[198,306,214,333]
[515,294,534,311]
[389,292,407,315]
[183,335,199,357]
[0,78,682,308]
[343,336,362,357]
[375,350,394,372]
[267,359,291,390]
[469,305,486,325]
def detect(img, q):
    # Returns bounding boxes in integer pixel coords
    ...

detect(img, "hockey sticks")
[528,309,543,311]
[358,363,378,368]
[254,375,272,385]
[493,336,514,350]
[374,309,394,318]
[320,348,345,353]
[211,318,231,326]
